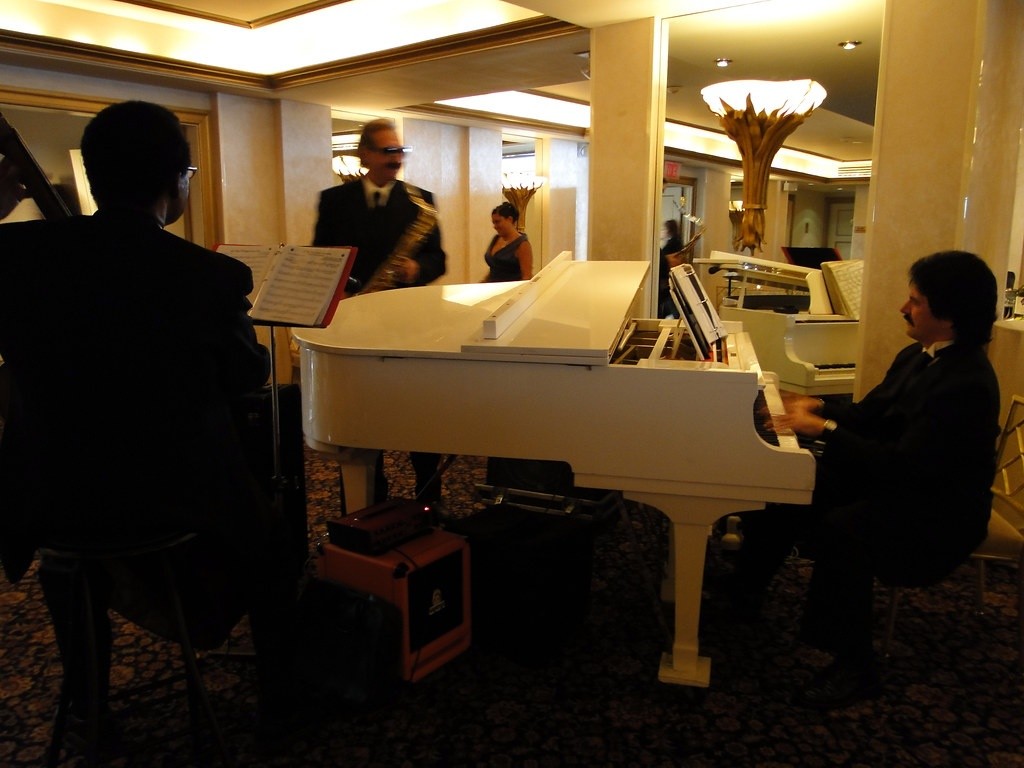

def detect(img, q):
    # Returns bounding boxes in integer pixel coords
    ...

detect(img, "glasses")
[365,145,405,154]
[185,166,198,179]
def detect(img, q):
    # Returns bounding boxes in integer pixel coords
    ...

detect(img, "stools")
[38,529,229,768]
[882,508,1024,657]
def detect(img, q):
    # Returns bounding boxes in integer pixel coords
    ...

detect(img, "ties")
[371,191,384,215]
[885,347,955,396]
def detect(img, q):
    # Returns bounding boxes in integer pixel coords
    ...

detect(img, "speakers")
[317,530,471,684]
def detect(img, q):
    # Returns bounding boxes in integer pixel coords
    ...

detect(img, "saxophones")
[351,176,446,301]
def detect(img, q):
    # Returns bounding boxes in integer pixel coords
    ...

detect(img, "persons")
[702,253,1001,685]
[485,203,531,282]
[659,220,683,279]
[311,121,456,527]
[0,101,364,753]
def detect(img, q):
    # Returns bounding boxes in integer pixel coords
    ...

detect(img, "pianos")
[687,247,869,402]
[286,247,823,694]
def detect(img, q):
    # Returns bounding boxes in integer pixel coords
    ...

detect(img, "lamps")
[332,155,369,183]
[700,79,827,256]
[502,172,547,233]
